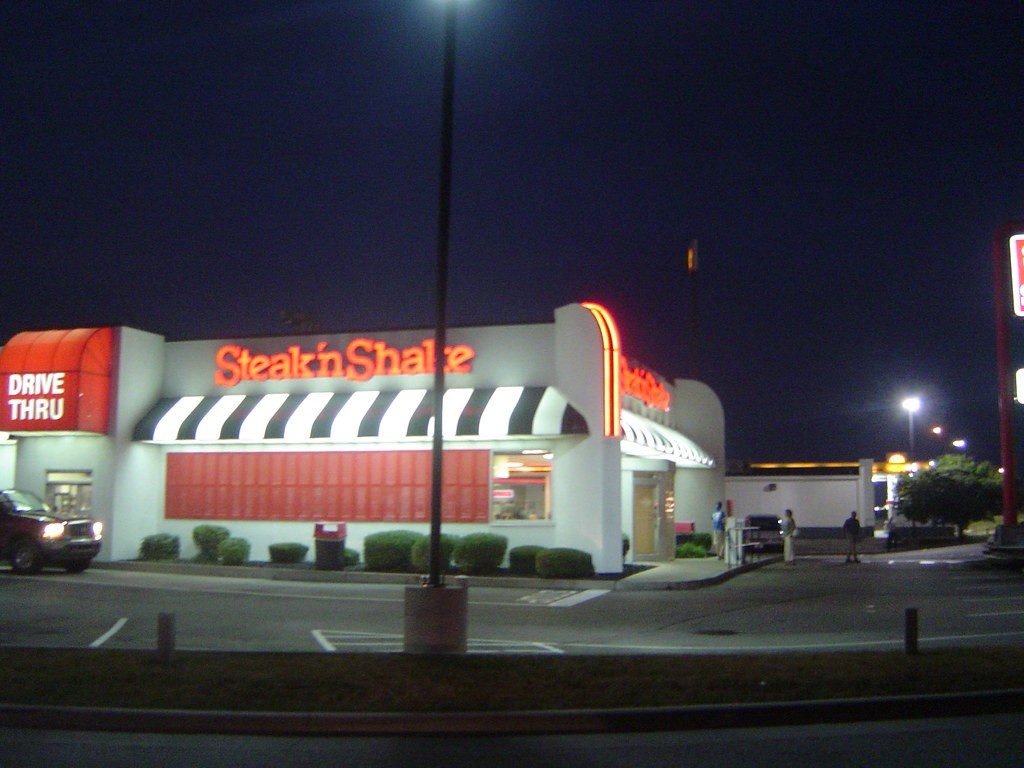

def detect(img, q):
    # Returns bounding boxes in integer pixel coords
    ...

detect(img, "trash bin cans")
[314,522,348,571]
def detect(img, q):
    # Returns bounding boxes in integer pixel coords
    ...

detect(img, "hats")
[715,502,722,511]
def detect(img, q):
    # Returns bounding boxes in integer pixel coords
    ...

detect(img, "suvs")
[0,489,103,575]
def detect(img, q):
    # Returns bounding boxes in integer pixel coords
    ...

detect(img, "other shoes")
[845,558,853,562]
[853,560,862,563]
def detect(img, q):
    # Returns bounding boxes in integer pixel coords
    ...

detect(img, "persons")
[783,510,796,565]
[711,501,726,559]
[843,512,861,564]
[501,495,520,519]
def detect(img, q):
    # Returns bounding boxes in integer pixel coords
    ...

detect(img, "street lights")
[900,394,923,465]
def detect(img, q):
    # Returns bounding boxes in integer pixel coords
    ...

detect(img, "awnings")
[0,331,109,435]
[134,386,590,445]
[620,412,717,468]
